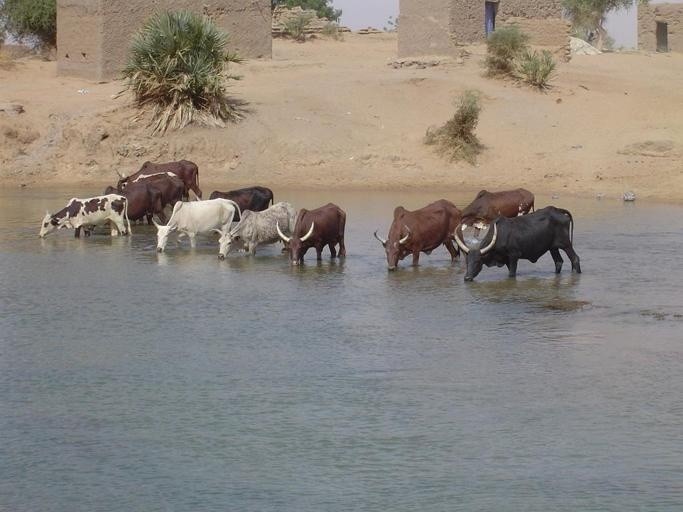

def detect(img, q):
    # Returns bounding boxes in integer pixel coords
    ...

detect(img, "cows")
[196,185,274,222]
[37,194,132,240]
[211,201,297,261]
[374,199,462,272]
[453,187,581,283]
[91,159,203,233]
[276,202,347,266]
[151,197,242,253]
